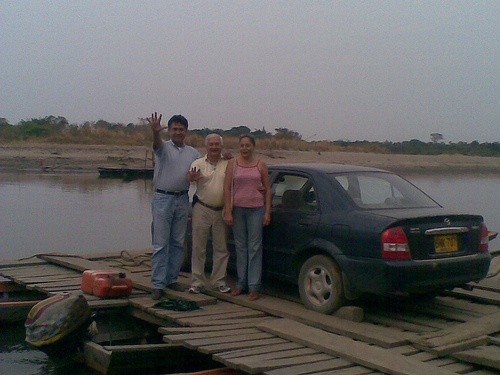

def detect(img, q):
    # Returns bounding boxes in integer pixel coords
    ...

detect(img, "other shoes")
[189,286,203,294]
[231,288,247,296]
[151,289,160,300]
[170,282,186,292]
[247,292,259,301]
[212,287,231,293]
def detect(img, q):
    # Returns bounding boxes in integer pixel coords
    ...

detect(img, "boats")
[96,167,154,180]
[0,280,246,375]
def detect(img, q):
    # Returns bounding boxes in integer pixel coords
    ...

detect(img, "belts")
[197,199,223,211]
[157,189,188,196]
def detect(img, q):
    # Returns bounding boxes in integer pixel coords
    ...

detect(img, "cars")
[151,162,492,315]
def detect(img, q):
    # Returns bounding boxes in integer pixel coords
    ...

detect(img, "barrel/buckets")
[81,268,133,299]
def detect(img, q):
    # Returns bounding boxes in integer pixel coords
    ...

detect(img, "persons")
[224,135,272,301]
[188,134,265,295]
[146,112,234,300]
